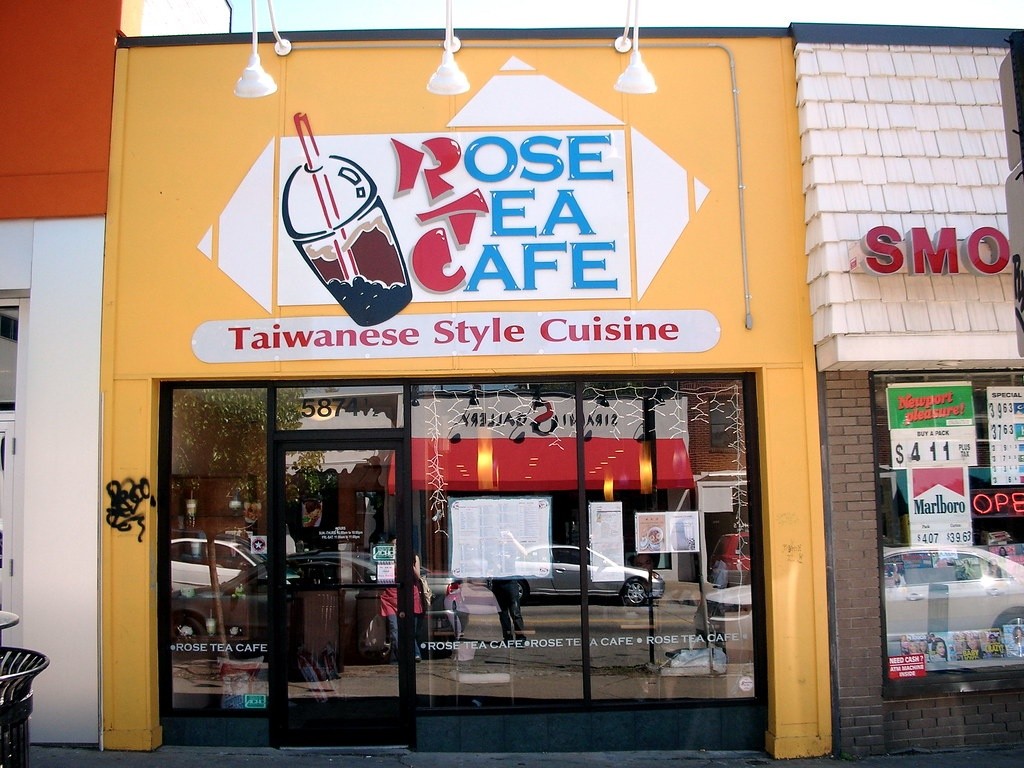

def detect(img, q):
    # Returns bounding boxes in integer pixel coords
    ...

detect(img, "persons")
[989,633,999,643]
[1012,626,1024,657]
[901,635,929,655]
[999,547,1008,556]
[987,559,1002,578]
[893,565,901,587]
[936,558,955,568]
[380,535,422,662]
[485,533,526,645]
[965,633,983,659]
[929,634,948,662]
[962,560,976,579]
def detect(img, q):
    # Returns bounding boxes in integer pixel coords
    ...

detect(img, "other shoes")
[515,636,526,649]
[498,638,514,646]
[416,656,421,663]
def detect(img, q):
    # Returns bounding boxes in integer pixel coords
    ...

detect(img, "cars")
[488,546,665,606]
[169,537,268,593]
[171,551,471,662]
[694,532,1024,662]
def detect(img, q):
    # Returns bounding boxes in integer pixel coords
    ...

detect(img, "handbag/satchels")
[416,576,432,609]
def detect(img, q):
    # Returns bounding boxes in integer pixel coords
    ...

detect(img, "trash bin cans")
[354,590,387,660]
[0,610,50,767]
[288,562,347,680]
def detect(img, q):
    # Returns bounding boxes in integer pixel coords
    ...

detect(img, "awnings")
[285,394,396,474]
[386,395,695,495]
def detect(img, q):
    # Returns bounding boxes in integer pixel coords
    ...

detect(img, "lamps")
[426,0,469,97]
[234,0,293,100]
[613,1,659,94]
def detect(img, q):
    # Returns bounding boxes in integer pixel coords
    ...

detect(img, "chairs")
[426,606,515,707]
